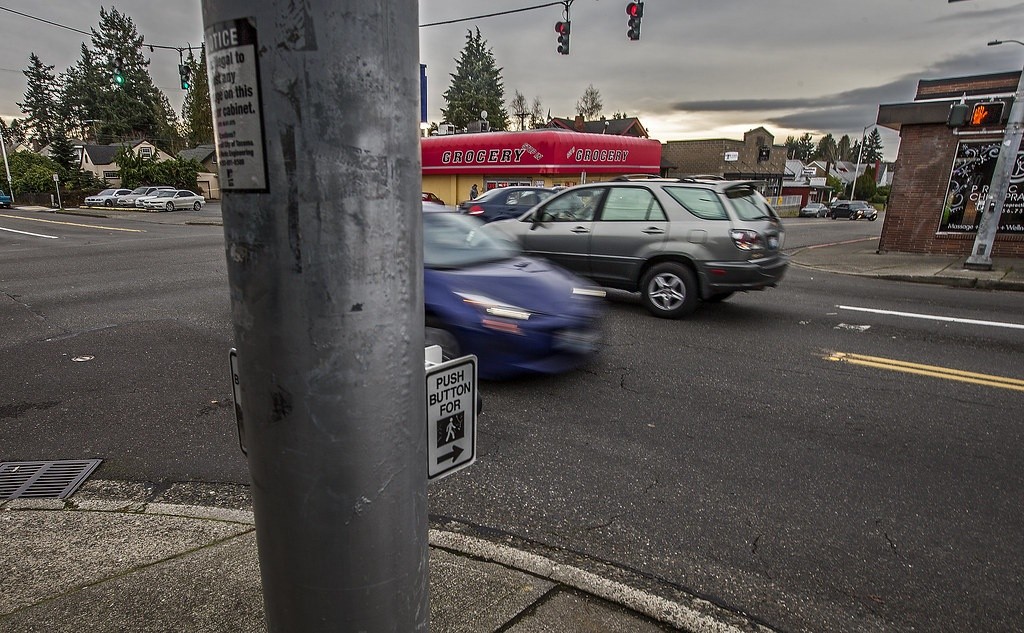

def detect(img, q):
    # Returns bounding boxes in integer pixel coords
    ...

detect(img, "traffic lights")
[970,103,1005,126]
[111,57,124,85]
[179,65,191,90]
[555,20,571,55]
[626,2,645,41]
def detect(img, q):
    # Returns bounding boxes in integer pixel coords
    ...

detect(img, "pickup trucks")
[0,190,11,208]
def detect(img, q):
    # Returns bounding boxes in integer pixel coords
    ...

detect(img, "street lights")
[850,123,877,201]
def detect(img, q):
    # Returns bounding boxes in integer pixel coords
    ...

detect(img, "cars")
[424,202,605,381]
[84,188,206,211]
[422,192,445,205]
[459,178,559,223]
[801,200,878,222]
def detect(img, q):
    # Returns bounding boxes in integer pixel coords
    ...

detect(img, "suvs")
[476,173,787,319]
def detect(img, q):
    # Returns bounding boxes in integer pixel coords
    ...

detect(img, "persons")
[470,183,478,200]
